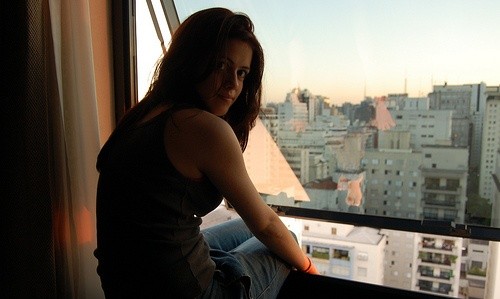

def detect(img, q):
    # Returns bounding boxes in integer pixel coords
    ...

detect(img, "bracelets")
[305,256,314,272]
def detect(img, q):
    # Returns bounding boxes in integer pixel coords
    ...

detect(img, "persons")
[93,8,321,299]
